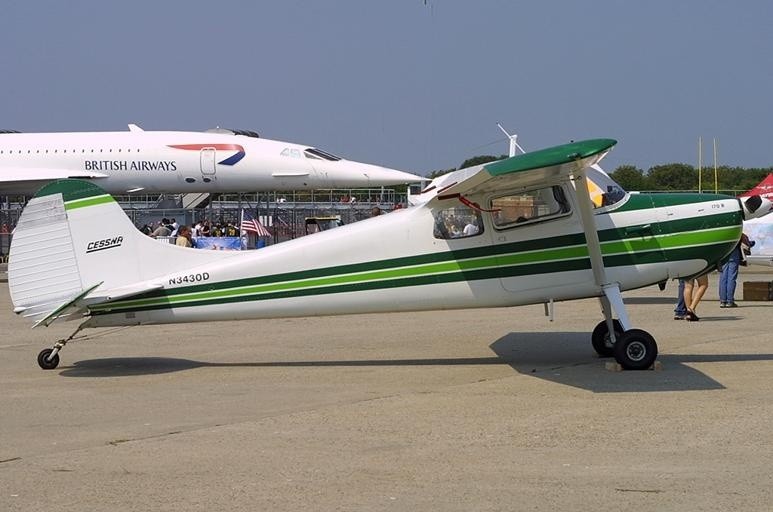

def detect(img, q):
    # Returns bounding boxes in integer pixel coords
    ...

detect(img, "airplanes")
[1,120,433,200]
[8,124,773,371]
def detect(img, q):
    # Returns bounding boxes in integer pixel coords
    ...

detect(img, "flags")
[242,208,272,238]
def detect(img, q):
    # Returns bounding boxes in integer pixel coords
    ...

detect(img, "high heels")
[686,307,699,321]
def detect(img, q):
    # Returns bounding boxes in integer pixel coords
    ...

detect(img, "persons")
[718,233,755,307]
[435,214,479,239]
[140,218,240,248]
[683,275,708,321]
[674,279,687,320]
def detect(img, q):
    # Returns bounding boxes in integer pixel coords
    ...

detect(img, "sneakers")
[674,313,686,319]
[720,302,738,308]
